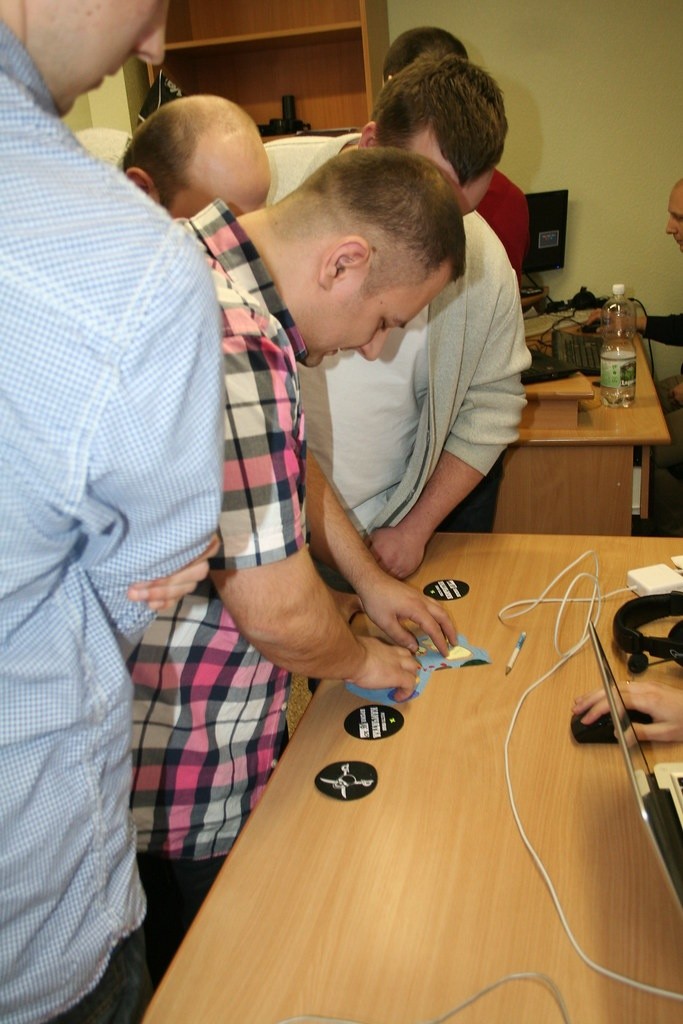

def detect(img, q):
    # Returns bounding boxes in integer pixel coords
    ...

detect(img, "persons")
[571,680,683,748]
[120,93,276,226]
[585,178,683,537]
[379,24,530,284]
[123,144,469,1016]
[0,0,222,1024]
[239,47,535,592]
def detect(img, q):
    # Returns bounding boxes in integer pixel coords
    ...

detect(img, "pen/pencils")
[504,632,527,675]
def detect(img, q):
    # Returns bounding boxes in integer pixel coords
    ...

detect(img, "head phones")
[613,590,683,669]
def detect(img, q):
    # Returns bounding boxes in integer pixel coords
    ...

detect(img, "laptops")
[589,620,683,911]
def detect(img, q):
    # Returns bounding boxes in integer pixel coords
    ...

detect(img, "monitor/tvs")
[522,189,568,274]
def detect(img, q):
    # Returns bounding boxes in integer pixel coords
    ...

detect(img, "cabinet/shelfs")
[147,0,390,146]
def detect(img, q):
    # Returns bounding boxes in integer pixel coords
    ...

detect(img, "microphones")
[627,653,673,673]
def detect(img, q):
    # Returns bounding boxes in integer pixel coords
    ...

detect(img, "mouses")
[582,320,601,333]
[571,705,653,744]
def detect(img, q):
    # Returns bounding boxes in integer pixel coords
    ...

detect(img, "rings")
[627,681,629,684]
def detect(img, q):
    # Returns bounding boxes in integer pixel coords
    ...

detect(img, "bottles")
[600,283,636,407]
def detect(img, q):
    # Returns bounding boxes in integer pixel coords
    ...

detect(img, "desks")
[488,325,671,533]
[143,531,683,1024]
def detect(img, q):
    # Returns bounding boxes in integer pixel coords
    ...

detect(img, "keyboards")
[524,307,601,337]
[551,329,605,376]
[520,349,576,385]
